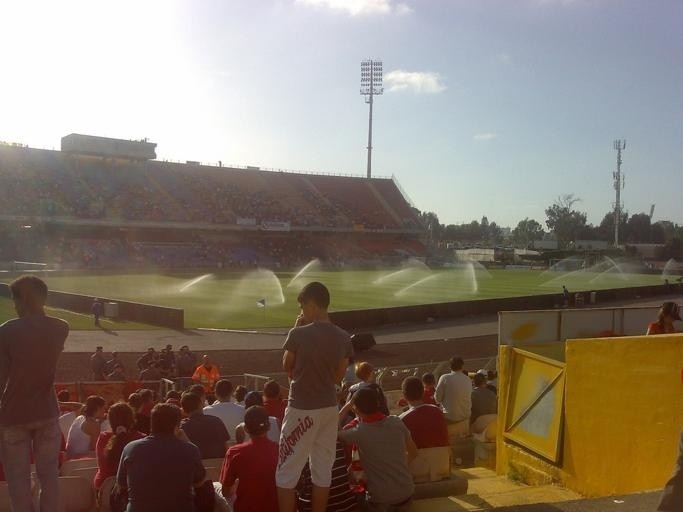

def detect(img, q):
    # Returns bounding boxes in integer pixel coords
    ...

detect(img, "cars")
[446,240,513,249]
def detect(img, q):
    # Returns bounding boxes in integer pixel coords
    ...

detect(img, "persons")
[0,142,434,270]
[297,356,498,512]
[645,302,683,335]
[275,283,355,512]
[0,275,70,512]
[59,346,287,512]
[560,285,570,307]
[90,297,103,327]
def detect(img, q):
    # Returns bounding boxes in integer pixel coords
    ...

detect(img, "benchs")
[0,149,433,270]
[1,362,498,510]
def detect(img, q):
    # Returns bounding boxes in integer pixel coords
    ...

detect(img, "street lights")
[359,56,382,178]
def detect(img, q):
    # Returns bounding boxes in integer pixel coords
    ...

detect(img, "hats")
[477,369,489,378]
[244,404,270,430]
